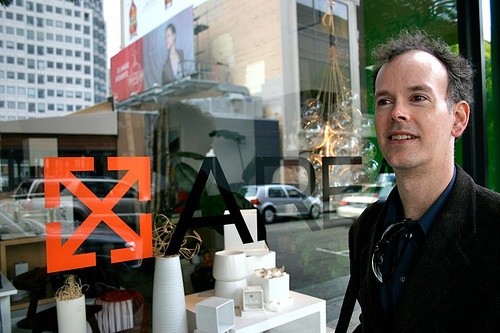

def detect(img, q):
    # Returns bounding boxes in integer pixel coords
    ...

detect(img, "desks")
[185,290,326,333]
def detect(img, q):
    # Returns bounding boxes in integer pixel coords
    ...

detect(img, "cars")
[336,173,396,222]
[237,184,323,225]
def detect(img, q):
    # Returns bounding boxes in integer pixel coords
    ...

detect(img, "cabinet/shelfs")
[0,107,146,311]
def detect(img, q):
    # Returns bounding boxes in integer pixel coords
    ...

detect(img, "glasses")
[371,219,410,282]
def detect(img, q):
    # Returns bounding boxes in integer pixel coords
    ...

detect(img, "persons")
[161,24,185,85]
[348,29,500,333]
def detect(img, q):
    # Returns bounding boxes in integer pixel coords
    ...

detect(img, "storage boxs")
[251,273,290,303]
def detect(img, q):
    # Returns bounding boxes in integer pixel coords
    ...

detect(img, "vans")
[10,175,139,229]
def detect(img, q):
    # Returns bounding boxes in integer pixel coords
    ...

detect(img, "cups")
[152,255,189,333]
